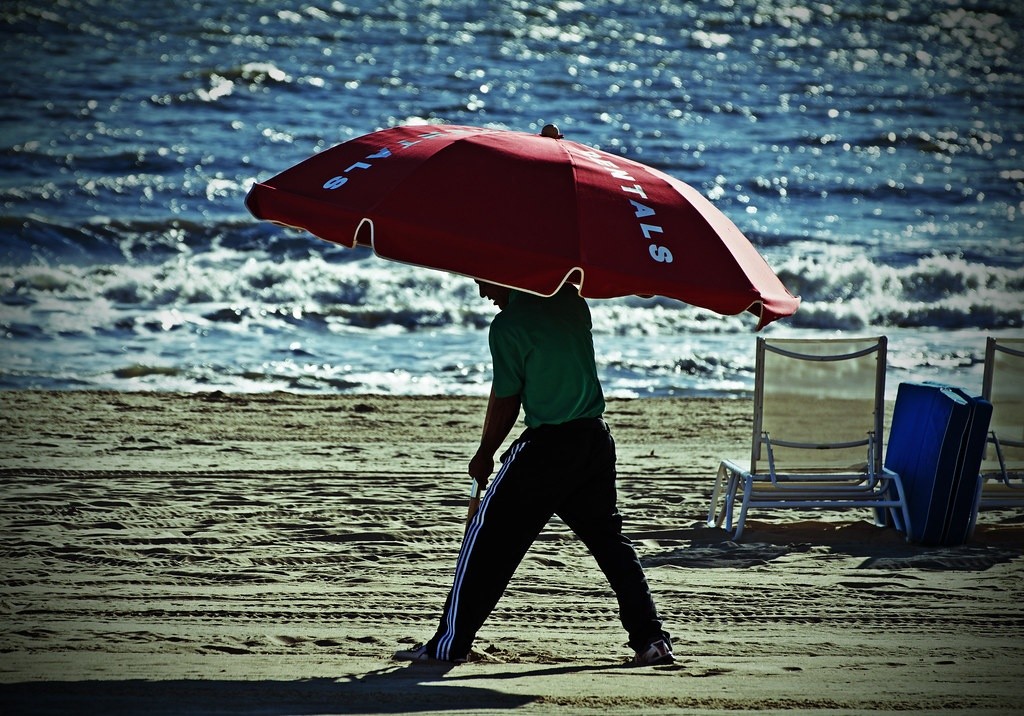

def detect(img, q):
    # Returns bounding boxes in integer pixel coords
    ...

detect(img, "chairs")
[977,337,1024,507]
[707,336,912,542]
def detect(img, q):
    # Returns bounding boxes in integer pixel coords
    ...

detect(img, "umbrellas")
[244,122,803,540]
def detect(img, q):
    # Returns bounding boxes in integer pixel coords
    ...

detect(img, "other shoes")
[395,645,469,662]
[633,639,676,665]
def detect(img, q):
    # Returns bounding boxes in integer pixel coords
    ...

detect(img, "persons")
[390,277,682,669]
